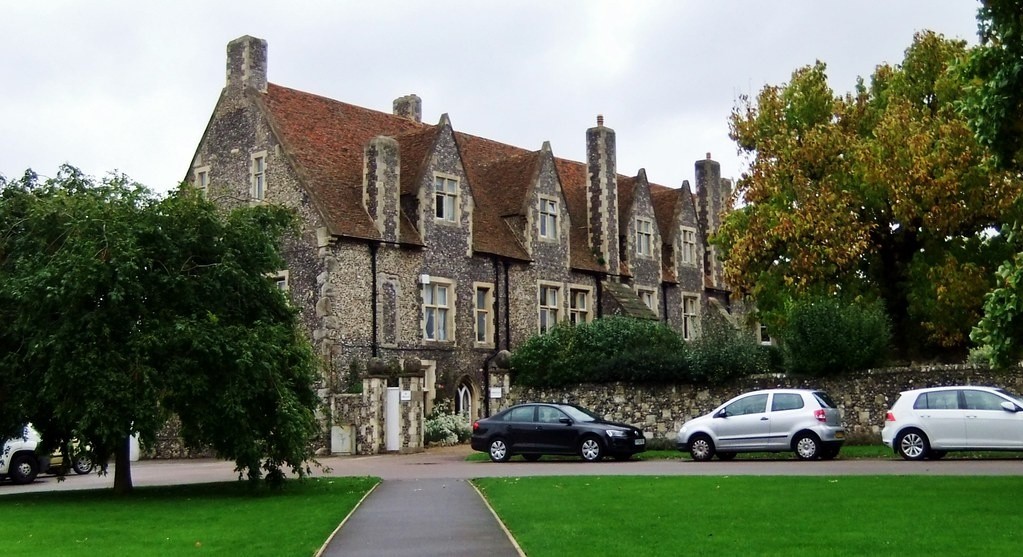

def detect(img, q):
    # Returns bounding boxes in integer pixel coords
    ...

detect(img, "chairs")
[512,408,558,423]
[967,395,988,410]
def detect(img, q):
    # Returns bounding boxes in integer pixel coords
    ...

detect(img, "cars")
[42,434,98,476]
[675,387,848,462]
[881,383,1023,461]
[0,424,46,486]
[471,400,649,464]
